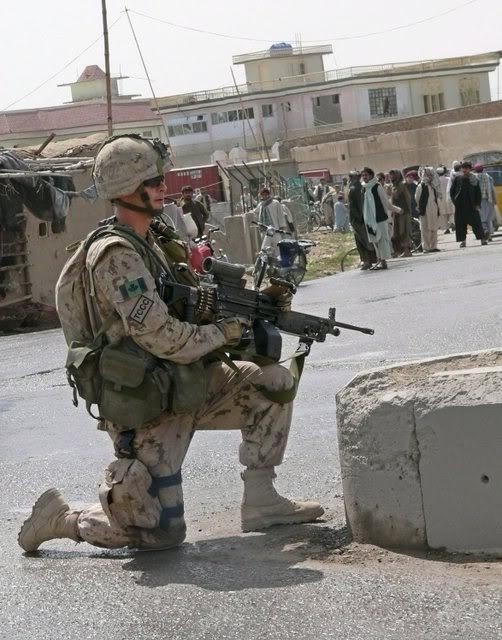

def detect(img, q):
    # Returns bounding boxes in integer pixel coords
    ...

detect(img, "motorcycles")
[248,221,315,291]
[182,228,229,277]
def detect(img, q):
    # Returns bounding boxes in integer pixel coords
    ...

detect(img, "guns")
[154,257,374,359]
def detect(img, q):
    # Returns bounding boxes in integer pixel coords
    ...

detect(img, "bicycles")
[337,217,424,273]
[307,200,324,231]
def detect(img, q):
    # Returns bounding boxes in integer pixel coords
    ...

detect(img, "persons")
[19,127,327,552]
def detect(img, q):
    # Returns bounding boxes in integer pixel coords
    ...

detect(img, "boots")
[239,469,324,531]
[17,490,84,552]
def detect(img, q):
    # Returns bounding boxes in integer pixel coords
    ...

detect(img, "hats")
[462,160,472,167]
[182,186,193,191]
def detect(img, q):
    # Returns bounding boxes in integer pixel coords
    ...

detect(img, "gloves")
[261,278,296,312]
[215,316,252,347]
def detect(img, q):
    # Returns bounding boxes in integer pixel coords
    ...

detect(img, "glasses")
[142,174,165,187]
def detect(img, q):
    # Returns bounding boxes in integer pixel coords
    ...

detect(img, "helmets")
[93,133,173,199]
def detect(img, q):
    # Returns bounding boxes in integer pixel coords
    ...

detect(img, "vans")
[465,161,502,232]
